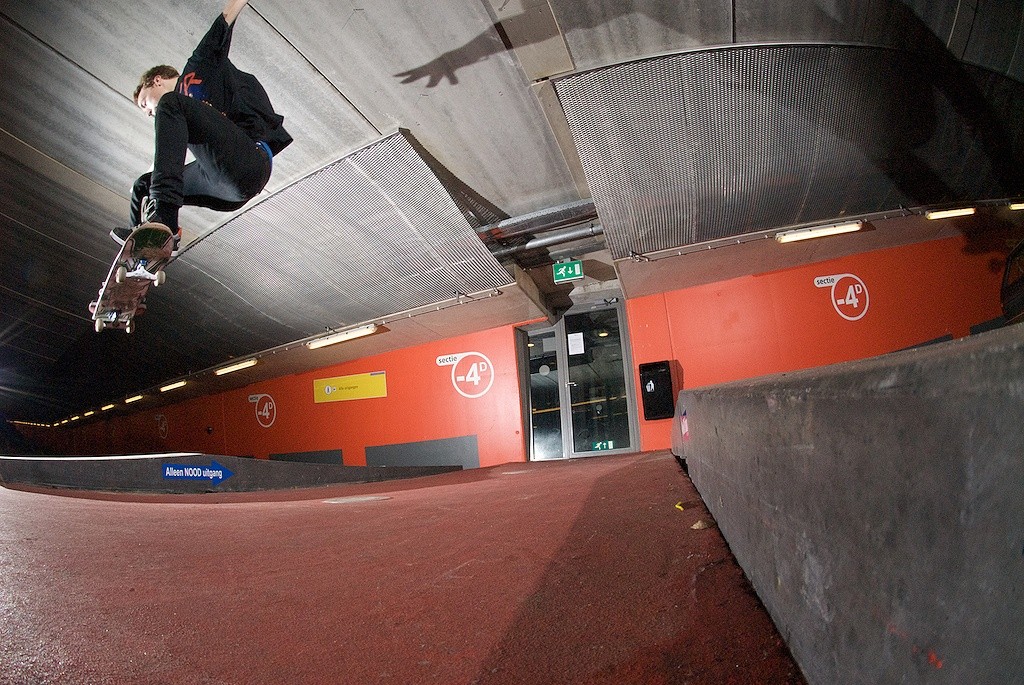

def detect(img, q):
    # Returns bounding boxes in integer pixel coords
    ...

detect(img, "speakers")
[638,361,674,420]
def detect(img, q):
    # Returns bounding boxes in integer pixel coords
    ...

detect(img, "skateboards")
[92,223,174,335]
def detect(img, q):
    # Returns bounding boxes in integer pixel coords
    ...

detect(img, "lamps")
[775,220,864,244]
[214,358,258,378]
[1007,201,1024,211]
[160,379,188,396]
[925,205,977,220]
[306,324,379,350]
[101,403,116,411]
[125,394,146,405]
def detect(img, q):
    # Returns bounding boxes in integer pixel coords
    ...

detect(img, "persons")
[109,0,293,257]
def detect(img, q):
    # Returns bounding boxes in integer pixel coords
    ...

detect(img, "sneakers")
[89,297,146,315]
[109,196,183,256]
